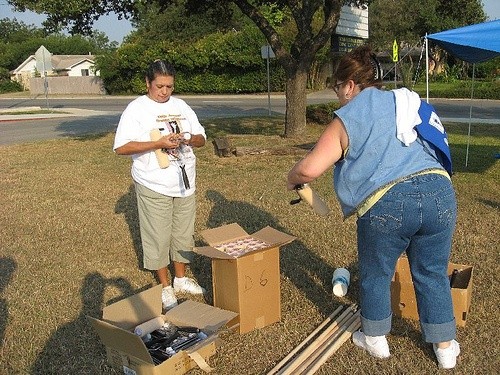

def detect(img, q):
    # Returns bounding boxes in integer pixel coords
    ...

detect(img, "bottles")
[289,183,330,217]
[134,317,164,337]
[149,130,169,168]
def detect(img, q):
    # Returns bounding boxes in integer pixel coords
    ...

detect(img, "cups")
[331,268,350,296]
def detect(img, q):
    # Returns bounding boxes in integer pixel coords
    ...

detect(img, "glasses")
[331,78,357,95]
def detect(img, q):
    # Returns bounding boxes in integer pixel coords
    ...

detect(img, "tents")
[380,20,500,170]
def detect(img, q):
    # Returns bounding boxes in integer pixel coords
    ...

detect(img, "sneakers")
[173,276,207,294]
[433,338,460,369]
[352,327,390,358]
[161,285,179,311]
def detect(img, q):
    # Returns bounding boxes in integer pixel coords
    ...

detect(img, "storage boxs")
[389,257,474,327]
[87,284,239,375]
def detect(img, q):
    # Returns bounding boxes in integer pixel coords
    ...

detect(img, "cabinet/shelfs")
[194,222,297,336]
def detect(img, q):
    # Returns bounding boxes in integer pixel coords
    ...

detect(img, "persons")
[286,45,460,369]
[112,59,207,315]
